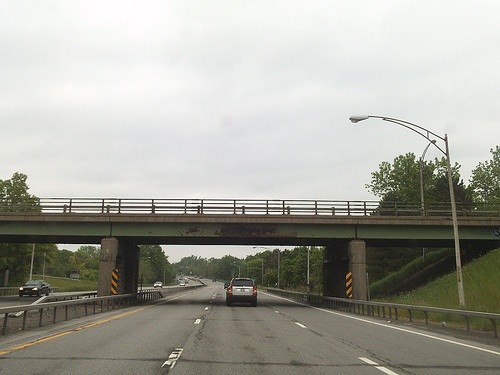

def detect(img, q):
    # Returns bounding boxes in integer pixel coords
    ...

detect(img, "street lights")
[233,262,249,278]
[229,263,241,278]
[420,139,438,261]
[253,247,273,287]
[246,254,263,287]
[348,114,466,311]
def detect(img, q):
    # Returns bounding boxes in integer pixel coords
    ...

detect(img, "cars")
[179,274,216,286]
[153,281,163,288]
[19,280,51,298]
[224,283,230,289]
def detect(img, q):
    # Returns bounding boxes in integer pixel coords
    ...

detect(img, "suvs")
[226,278,258,307]
